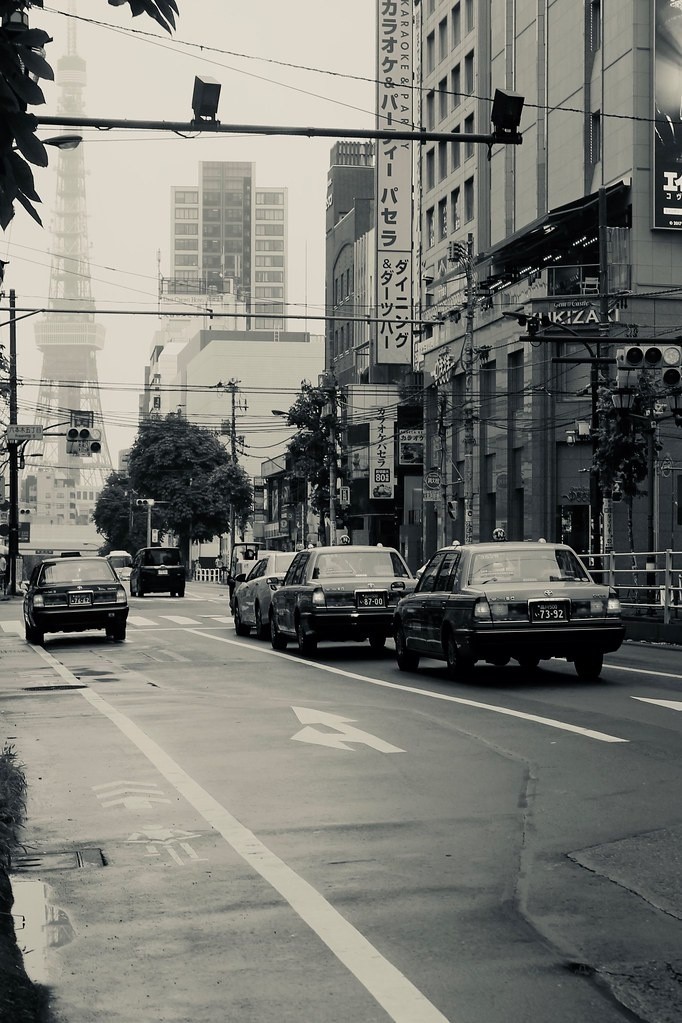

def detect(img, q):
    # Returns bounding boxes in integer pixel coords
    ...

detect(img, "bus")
[105,550,133,581]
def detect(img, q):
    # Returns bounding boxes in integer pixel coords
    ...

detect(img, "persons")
[215,554,224,580]
[194,559,202,571]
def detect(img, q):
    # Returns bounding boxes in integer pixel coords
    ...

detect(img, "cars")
[231,544,356,641]
[19,550,130,643]
[391,530,622,683]
[266,535,418,651]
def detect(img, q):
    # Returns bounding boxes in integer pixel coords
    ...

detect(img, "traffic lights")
[135,499,155,506]
[616,345,682,391]
[18,508,30,515]
[448,500,458,522]
[66,427,101,454]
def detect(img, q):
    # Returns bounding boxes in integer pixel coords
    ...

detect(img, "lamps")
[565,430,582,447]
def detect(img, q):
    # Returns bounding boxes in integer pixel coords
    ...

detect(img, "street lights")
[503,311,604,585]
[271,409,338,546]
[100,498,135,533]
[83,543,104,555]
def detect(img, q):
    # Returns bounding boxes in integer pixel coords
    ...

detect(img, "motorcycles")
[216,542,274,610]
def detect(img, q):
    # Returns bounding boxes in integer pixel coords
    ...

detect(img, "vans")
[130,547,186,598]
